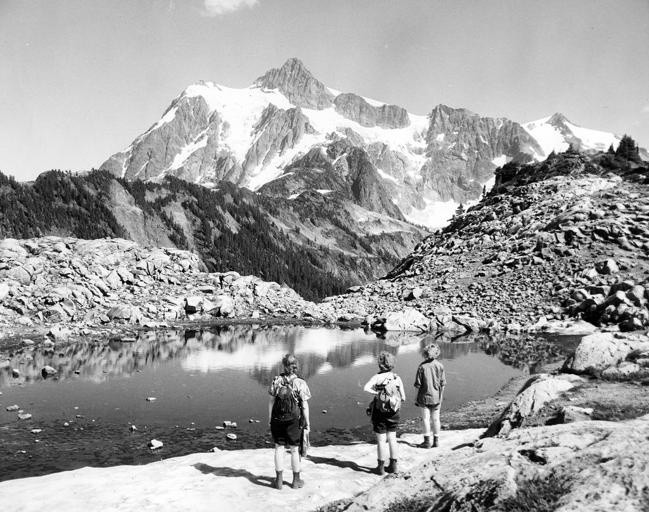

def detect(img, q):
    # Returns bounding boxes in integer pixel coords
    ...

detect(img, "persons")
[266,353,311,489]
[413,343,447,449]
[363,350,406,475]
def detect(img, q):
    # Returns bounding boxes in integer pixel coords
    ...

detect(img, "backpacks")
[273,373,300,422]
[378,374,401,413]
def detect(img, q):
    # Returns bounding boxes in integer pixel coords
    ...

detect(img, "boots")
[374,458,397,474]
[272,471,283,489]
[419,436,439,448]
[292,471,304,489]
